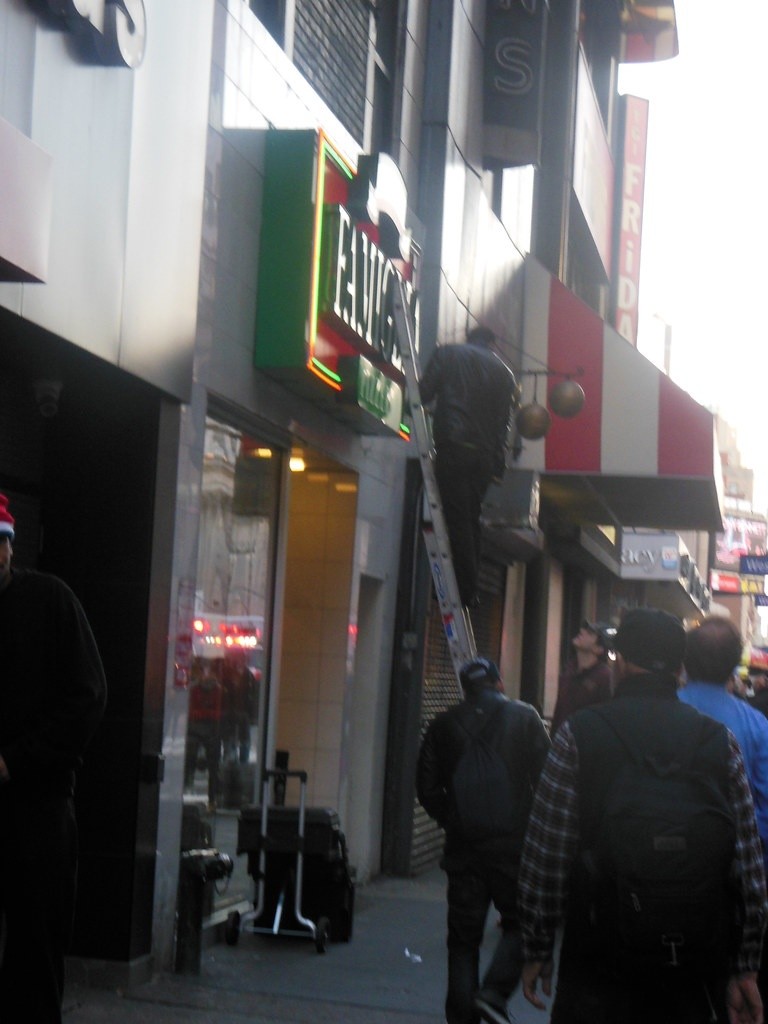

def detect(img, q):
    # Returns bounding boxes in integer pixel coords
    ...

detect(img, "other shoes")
[472,989,512,1024]
[208,801,215,813]
[432,586,480,607]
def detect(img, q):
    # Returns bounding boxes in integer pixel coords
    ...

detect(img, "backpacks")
[585,705,736,957]
[448,699,519,844]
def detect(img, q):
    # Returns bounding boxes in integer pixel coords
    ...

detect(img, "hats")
[0,493,15,542]
[459,657,499,677]
[616,606,686,674]
[583,617,618,652]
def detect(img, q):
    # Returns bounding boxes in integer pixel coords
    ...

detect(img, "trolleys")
[222,770,332,955]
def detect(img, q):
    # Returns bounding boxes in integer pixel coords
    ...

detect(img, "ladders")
[391,266,477,700]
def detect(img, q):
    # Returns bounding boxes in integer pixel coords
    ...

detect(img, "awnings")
[519,255,726,534]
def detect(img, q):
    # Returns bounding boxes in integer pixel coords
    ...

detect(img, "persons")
[417,660,551,1024]
[0,490,107,1024]
[728,674,768,719]
[676,618,768,865]
[406,328,515,604]
[518,610,768,1024]
[550,617,612,739]
[185,655,257,794]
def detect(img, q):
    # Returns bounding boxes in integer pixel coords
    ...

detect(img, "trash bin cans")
[178,794,214,929]
[242,805,341,943]
